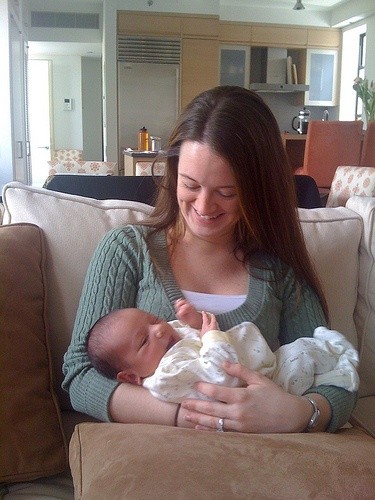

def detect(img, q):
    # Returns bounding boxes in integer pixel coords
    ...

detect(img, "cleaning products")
[137,125,148,152]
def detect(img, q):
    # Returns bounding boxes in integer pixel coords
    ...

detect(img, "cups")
[291,107,311,134]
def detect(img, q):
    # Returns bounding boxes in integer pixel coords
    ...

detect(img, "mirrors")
[117,62,181,174]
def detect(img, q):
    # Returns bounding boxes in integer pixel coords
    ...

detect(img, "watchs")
[302,395,321,433]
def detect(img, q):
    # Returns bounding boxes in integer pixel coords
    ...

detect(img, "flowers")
[353,78,375,121]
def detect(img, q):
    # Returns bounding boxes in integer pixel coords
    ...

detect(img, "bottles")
[150,136,162,152]
[322,108,329,121]
[138,126,149,152]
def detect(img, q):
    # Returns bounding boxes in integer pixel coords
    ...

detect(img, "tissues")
[149,136,161,152]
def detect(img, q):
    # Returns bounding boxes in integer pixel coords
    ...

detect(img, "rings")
[218,418,224,433]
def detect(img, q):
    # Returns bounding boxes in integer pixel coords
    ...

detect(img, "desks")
[121,150,167,176]
[281,133,363,152]
[43,173,321,209]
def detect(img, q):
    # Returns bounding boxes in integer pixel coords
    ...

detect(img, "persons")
[60,84,360,437]
[85,299,359,407]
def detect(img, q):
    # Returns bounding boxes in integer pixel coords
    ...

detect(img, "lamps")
[293,0,305,10]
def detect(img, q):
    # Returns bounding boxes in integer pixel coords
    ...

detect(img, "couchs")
[0,182,375,500]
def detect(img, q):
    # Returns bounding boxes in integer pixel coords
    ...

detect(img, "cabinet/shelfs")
[181,39,219,114]
[219,45,250,90]
[291,48,341,107]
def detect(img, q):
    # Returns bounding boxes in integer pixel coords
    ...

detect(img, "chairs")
[294,120,375,210]
[48,148,115,177]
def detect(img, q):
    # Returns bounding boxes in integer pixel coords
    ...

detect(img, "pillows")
[0,223,81,485]
[69,423,375,500]
